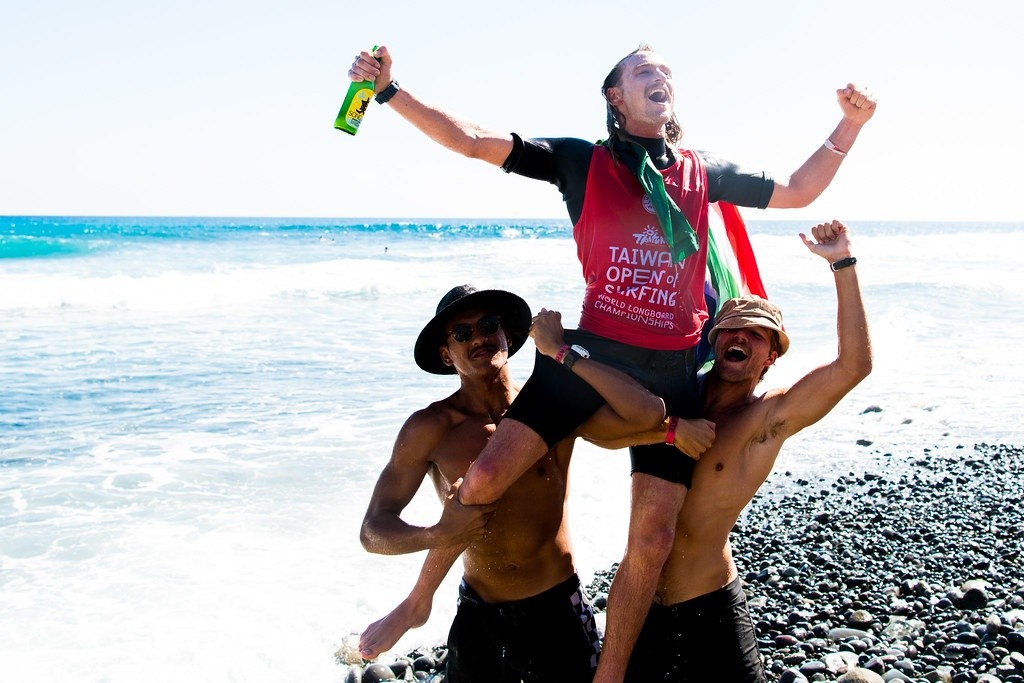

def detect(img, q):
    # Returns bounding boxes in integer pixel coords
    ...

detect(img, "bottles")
[333,45,383,135]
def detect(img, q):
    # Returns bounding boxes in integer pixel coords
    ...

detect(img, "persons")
[361,284,665,683]
[347,44,878,683]
[586,219,873,683]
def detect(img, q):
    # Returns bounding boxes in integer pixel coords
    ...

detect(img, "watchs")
[563,345,590,371]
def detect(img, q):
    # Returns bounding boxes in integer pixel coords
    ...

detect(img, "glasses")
[441,314,501,346]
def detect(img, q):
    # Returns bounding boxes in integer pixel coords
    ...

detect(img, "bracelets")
[824,139,848,157]
[831,257,857,272]
[556,344,570,364]
[665,416,680,446]
[374,79,399,104]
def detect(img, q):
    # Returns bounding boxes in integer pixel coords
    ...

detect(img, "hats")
[414,284,532,375]
[708,294,790,359]
